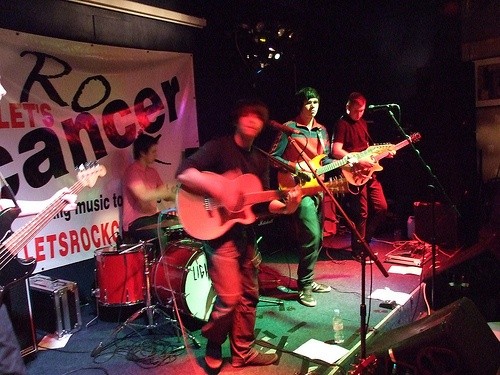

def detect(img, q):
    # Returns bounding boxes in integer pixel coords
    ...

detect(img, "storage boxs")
[28,274,83,341]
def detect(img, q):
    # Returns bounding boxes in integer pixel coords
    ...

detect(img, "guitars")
[0,160,108,292]
[277,153,357,201]
[341,132,423,186]
[176,171,351,240]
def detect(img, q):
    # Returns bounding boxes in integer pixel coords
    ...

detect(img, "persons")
[0,76,77,375]
[267,86,373,307]
[122,134,178,250]
[331,92,398,266]
[174,103,302,368]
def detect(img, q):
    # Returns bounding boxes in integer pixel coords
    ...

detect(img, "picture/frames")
[471,56,500,107]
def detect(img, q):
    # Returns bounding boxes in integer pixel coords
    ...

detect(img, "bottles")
[332,309,345,344]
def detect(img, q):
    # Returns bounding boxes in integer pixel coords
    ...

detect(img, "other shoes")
[205,339,222,369]
[245,353,279,365]
[373,251,378,255]
[352,252,374,263]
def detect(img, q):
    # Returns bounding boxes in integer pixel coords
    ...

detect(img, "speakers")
[353,231,500,375]
[3,277,42,359]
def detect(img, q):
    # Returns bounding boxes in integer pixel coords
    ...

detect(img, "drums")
[148,239,217,322]
[94,243,147,308]
[164,225,190,243]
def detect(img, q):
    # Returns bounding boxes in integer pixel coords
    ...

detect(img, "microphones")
[368,104,398,112]
[269,121,304,135]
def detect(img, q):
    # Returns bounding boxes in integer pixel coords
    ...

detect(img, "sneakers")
[310,281,330,293]
[297,289,316,306]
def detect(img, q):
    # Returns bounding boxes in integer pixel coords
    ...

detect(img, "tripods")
[90,242,201,357]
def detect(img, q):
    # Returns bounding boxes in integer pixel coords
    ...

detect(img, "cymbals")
[135,220,180,230]
